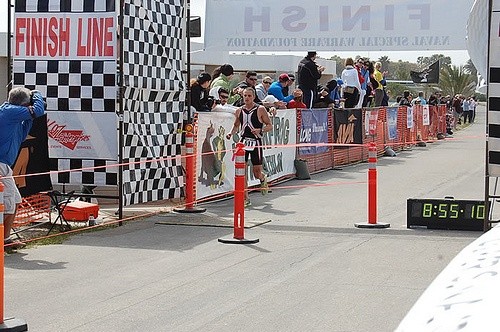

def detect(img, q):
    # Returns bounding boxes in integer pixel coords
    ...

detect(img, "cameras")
[29,96,47,111]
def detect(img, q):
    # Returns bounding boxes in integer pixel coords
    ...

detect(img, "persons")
[0,86,44,254]
[190,49,479,135]
[226,87,273,208]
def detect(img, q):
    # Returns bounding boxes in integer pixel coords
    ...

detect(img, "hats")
[279,73,290,82]
[307,50,317,54]
[434,91,442,94]
[288,74,296,80]
[265,95,279,104]
[222,65,233,76]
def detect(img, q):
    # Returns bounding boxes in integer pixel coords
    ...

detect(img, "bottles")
[89,214,94,226]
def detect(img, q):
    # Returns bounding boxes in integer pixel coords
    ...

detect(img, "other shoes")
[3,239,27,251]
[260,172,267,188]
[244,199,251,207]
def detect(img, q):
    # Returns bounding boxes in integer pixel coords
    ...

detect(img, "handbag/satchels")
[370,78,379,89]
[380,80,386,86]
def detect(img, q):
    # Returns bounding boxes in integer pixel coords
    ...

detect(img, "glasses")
[358,62,364,64]
[263,81,272,85]
[364,64,369,66]
[220,95,228,99]
[248,78,258,81]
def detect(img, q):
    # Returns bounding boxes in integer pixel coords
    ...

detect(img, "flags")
[410,60,439,84]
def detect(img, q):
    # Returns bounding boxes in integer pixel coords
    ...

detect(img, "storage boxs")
[60,201,99,221]
